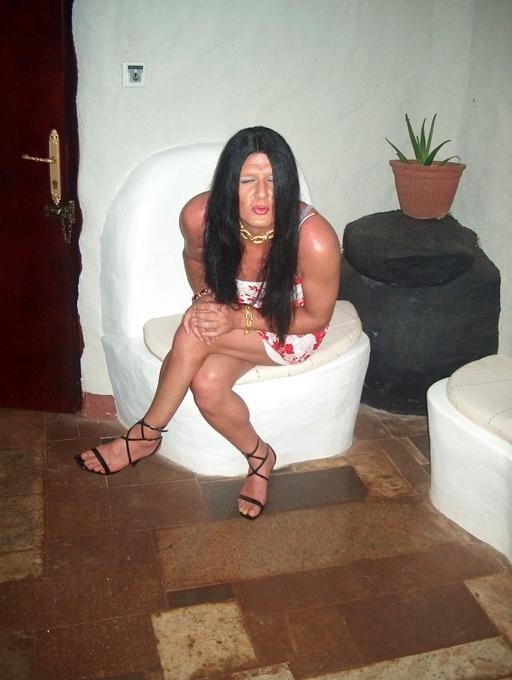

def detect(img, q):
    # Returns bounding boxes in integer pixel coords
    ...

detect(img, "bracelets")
[191,287,217,304]
[244,305,255,335]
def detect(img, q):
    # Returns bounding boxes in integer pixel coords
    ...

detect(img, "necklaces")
[239,222,276,244]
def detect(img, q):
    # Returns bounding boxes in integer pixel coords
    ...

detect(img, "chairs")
[425,351,512,569]
[98,140,373,480]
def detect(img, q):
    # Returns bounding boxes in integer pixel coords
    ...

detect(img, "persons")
[74,126,340,520]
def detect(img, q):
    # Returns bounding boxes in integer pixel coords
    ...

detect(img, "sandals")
[75,418,168,475]
[238,437,276,520]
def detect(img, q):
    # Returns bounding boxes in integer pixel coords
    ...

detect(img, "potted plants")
[382,110,469,222]
